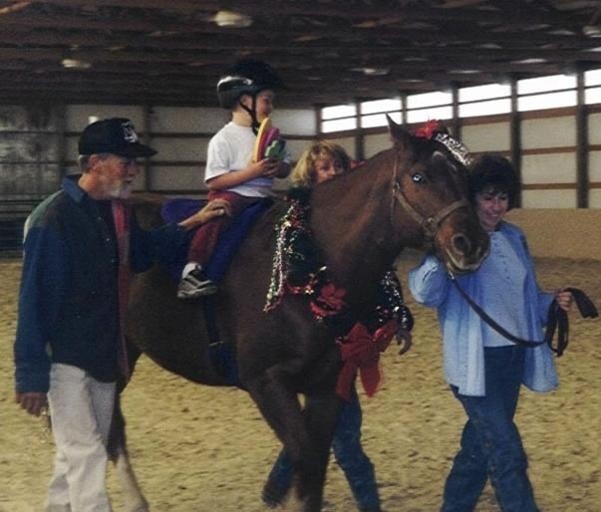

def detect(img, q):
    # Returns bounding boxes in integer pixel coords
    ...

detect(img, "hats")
[78,118,158,157]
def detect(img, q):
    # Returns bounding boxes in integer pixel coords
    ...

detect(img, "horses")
[43,111,493,512]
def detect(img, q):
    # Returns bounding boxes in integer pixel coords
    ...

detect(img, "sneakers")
[177,270,217,298]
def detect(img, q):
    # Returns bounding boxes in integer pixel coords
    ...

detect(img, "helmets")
[217,62,283,95]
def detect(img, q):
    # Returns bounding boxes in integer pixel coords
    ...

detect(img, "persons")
[14,115,232,512]
[408,155,574,511]
[262,140,415,512]
[175,68,293,299]
